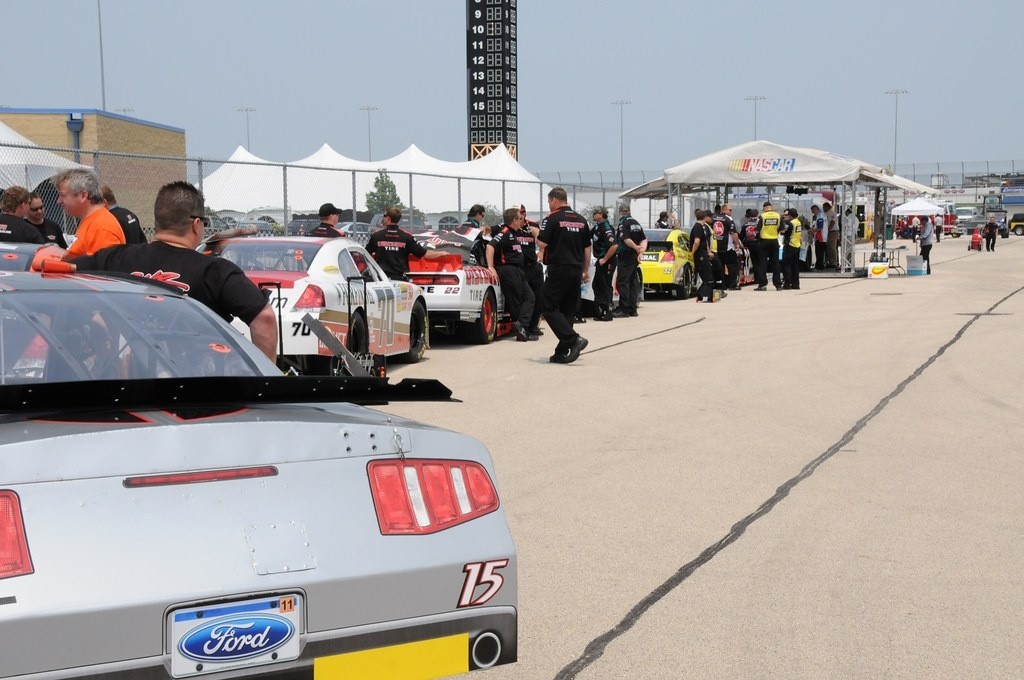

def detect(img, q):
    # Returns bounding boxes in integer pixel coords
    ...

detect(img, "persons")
[808,202,860,273]
[65,181,278,366]
[611,203,648,317]
[24,193,68,249]
[300,203,346,266]
[51,167,126,262]
[365,206,449,282]
[985,217,998,252]
[741,202,801,291]
[590,205,619,321]
[0,186,45,245]
[654,211,678,229]
[530,187,592,363]
[460,204,544,342]
[920,216,933,260]
[896,215,942,242]
[99,183,148,245]
[689,203,741,304]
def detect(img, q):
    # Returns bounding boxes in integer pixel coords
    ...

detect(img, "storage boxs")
[905,255,928,275]
[867,261,891,279]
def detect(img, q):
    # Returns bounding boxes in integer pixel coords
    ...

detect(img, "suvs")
[955,206,981,224]
[281,219,320,236]
[1008,212,1024,236]
[369,214,433,234]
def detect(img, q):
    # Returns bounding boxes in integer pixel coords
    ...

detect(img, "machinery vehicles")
[980,194,1010,238]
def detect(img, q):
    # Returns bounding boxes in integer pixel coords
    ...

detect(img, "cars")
[634,229,700,298]
[198,225,434,378]
[1,268,522,678]
[235,221,278,238]
[338,221,372,237]
[204,216,232,236]
[406,228,515,344]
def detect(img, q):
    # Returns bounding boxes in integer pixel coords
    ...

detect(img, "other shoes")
[753,285,767,291]
[512,321,528,341]
[774,283,781,290]
[612,308,630,318]
[573,316,586,323]
[630,309,638,316]
[593,311,612,321]
[529,327,544,335]
[714,284,742,297]
[517,335,539,341]
[782,283,801,289]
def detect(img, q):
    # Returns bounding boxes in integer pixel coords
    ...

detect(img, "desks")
[851,247,910,276]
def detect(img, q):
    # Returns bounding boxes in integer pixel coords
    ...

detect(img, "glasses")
[30,205,43,212]
[592,211,602,216]
[191,212,210,227]
[783,214,790,218]
[383,215,388,217]
[480,213,484,218]
[725,208,732,212]
[520,210,526,214]
[20,200,32,206]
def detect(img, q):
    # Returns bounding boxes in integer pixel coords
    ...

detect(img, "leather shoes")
[550,353,567,362]
[565,335,588,362]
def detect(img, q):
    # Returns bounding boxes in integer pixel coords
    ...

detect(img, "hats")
[319,203,342,218]
[762,202,771,211]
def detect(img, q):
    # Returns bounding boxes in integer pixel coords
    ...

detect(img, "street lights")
[743,94,767,139]
[886,87,910,173]
[359,105,379,162]
[611,99,633,170]
[236,106,256,153]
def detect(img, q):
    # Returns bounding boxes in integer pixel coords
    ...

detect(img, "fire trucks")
[895,200,966,238]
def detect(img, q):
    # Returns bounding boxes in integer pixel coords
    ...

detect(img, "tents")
[192,143,590,230]
[890,197,944,237]
[0,120,94,192]
[618,139,943,201]
